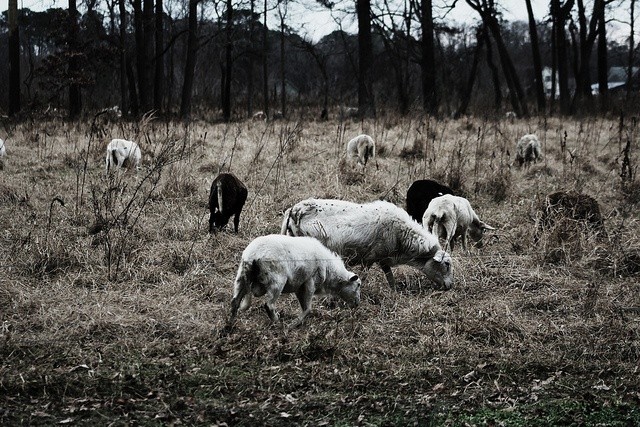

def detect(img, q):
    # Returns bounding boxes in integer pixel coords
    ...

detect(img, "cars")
[591,66,640,100]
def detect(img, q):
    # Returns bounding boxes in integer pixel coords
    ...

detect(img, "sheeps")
[209,171,248,234]
[515,134,541,170]
[421,194,498,257]
[279,198,453,310]
[343,134,376,165]
[105,138,142,176]
[405,179,467,225]
[222,233,362,342]
